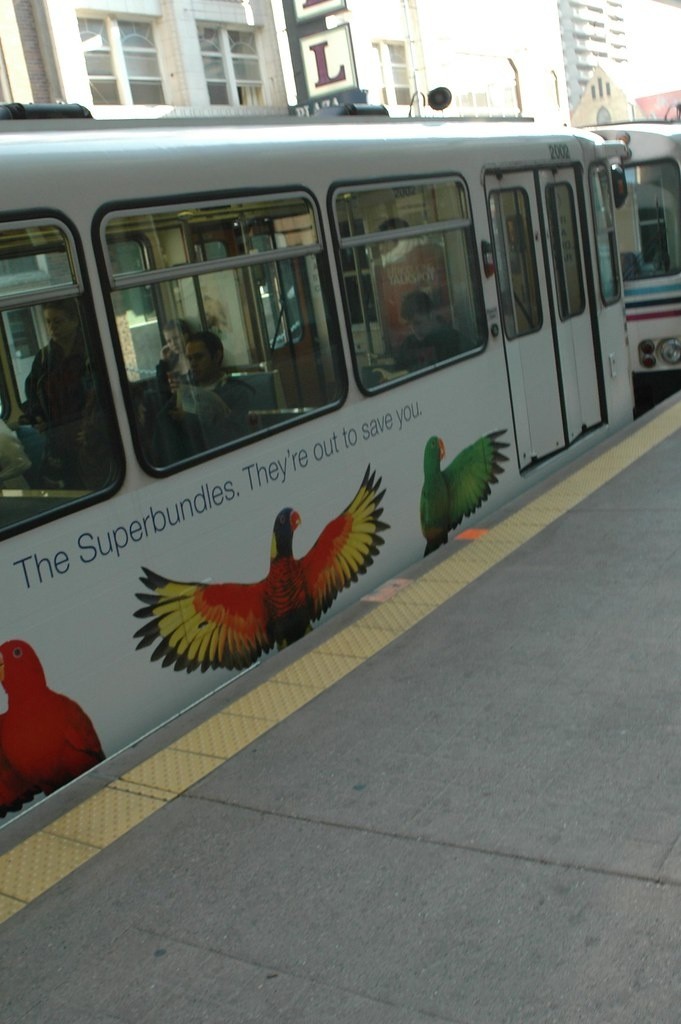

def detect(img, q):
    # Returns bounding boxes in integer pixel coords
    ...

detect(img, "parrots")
[0,640,106,822]
[417,429,512,556]
[133,461,391,673]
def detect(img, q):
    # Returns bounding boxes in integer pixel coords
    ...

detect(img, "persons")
[377,217,446,366]
[395,291,465,371]
[140,318,250,463]
[16,299,118,493]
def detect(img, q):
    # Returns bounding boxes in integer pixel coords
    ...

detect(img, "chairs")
[619,251,642,279]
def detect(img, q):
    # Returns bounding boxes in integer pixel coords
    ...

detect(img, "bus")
[1,103,681,858]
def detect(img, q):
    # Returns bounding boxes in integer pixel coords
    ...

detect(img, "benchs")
[140,370,286,411]
[243,406,309,430]
[12,424,46,485]
